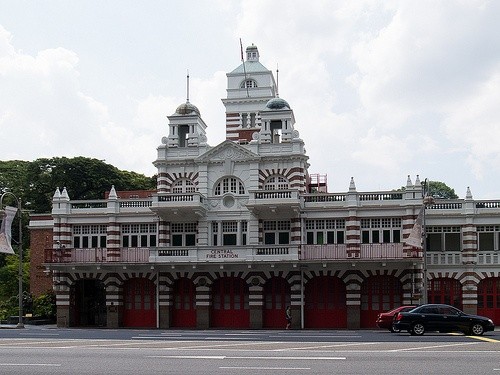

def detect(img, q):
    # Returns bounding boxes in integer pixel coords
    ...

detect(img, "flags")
[0,206,18,254]
[240,40,243,60]
[405,205,424,249]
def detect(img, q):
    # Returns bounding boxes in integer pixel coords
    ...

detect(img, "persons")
[286,306,292,329]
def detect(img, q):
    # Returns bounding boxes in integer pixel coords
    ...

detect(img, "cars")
[376,303,495,336]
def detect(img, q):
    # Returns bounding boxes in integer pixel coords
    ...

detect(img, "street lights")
[0,191,24,329]
[421,177,436,304]
